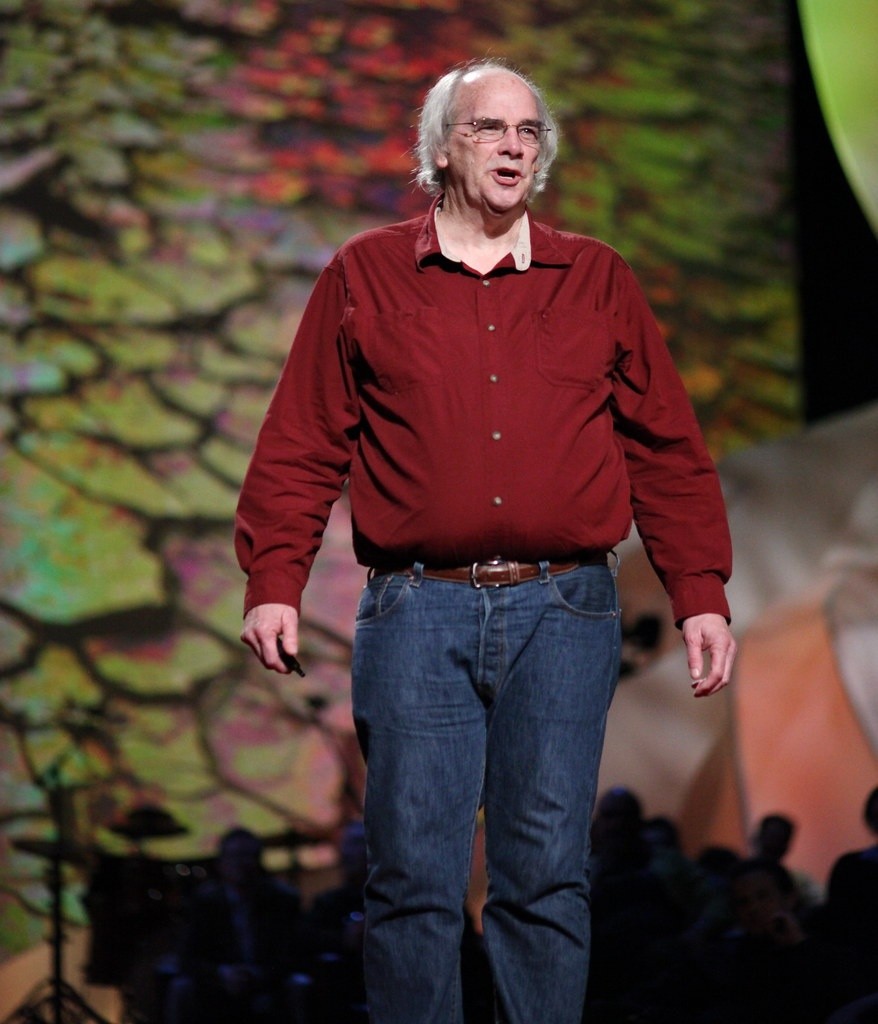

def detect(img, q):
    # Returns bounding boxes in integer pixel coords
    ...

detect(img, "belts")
[395,549,609,589]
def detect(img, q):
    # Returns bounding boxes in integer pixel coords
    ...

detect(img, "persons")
[234,57,738,1024]
[128,782,878,1023]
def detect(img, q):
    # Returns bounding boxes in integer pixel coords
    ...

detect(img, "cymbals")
[262,833,322,846]
[10,838,74,861]
[105,820,186,839]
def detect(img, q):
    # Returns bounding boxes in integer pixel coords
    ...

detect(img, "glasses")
[446,116,551,144]
